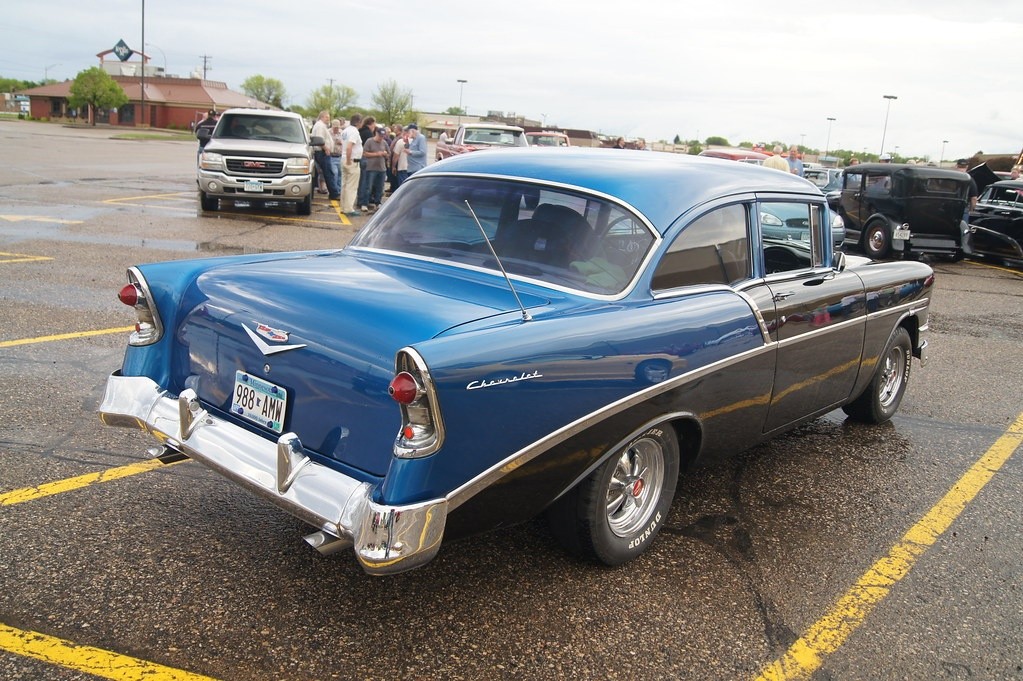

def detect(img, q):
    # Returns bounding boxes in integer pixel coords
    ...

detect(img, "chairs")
[229,125,249,137]
[499,219,570,270]
[684,243,740,285]
[532,204,606,262]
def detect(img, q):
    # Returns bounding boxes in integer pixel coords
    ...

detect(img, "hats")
[958,158,968,165]
[404,124,417,131]
[878,153,890,160]
[376,128,387,137]
[208,109,216,115]
[332,119,340,129]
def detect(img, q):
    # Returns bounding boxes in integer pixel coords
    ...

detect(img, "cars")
[968,171,1023,268]
[802,163,857,195]
[196,109,325,216]
[758,202,846,248]
[697,149,770,167]
[98,145,935,576]
[820,162,971,259]
[525,132,570,148]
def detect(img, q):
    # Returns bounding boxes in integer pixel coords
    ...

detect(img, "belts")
[332,155,340,157]
[350,159,361,162]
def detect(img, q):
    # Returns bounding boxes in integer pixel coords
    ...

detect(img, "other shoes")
[330,194,341,200]
[361,204,369,212]
[385,194,392,197]
[318,189,328,194]
[343,211,361,217]
[374,203,381,210]
[385,189,391,192]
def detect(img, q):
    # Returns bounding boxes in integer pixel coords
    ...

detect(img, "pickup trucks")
[435,123,540,209]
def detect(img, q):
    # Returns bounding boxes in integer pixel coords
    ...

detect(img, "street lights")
[941,141,948,162]
[457,80,468,124]
[881,96,897,154]
[825,118,836,160]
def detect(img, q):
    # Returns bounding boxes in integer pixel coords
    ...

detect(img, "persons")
[784,146,803,177]
[1010,168,1020,180]
[763,146,790,172]
[638,138,651,150]
[440,129,452,140]
[869,154,892,187]
[195,109,218,166]
[613,136,625,149]
[838,158,861,190]
[956,158,978,224]
[312,110,428,216]
